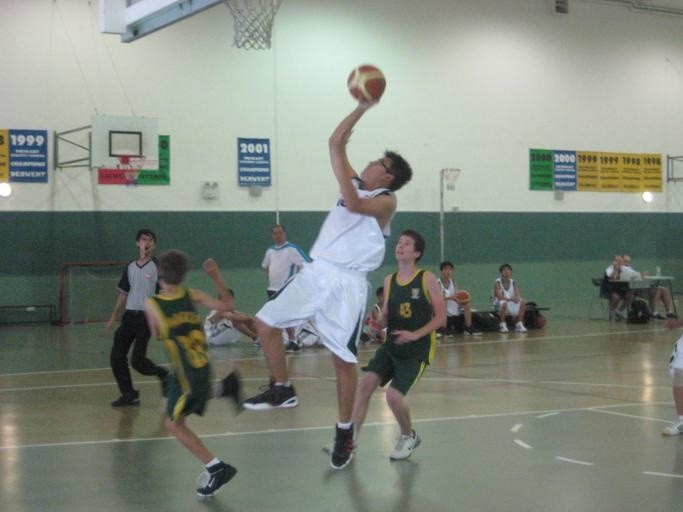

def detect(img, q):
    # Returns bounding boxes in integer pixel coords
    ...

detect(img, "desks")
[609,275,678,324]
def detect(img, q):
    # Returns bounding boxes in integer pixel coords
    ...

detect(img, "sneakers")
[112,390,140,406]
[464,327,482,336]
[287,340,302,351]
[663,419,682,435]
[241,375,298,410]
[254,338,260,349]
[331,422,355,469]
[442,329,455,336]
[610,313,621,321]
[390,429,420,459]
[196,461,237,497]
[499,322,508,332]
[667,312,678,318]
[515,321,528,332]
[652,314,664,320]
[160,368,171,396]
[221,370,241,412]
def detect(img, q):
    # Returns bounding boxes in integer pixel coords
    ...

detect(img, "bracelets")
[614,267,619,272]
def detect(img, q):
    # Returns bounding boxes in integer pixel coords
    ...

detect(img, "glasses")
[378,158,392,174]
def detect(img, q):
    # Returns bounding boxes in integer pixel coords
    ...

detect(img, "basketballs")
[348,66,385,103]
[456,290,470,306]
[536,314,545,327]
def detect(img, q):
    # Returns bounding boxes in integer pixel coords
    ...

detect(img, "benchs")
[439,305,551,336]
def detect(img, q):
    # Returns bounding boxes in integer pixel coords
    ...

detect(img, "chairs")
[630,292,669,322]
[589,277,628,320]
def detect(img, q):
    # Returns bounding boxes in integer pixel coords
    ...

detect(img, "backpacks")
[523,302,546,328]
[627,301,651,323]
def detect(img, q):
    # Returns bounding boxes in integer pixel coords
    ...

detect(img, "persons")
[435,261,483,338]
[662,318,683,437]
[107,228,172,407]
[600,255,640,322]
[142,248,240,497]
[241,99,411,470]
[490,264,527,334]
[369,286,388,344]
[621,255,680,321]
[320,231,448,462]
[261,223,313,354]
[204,289,263,347]
